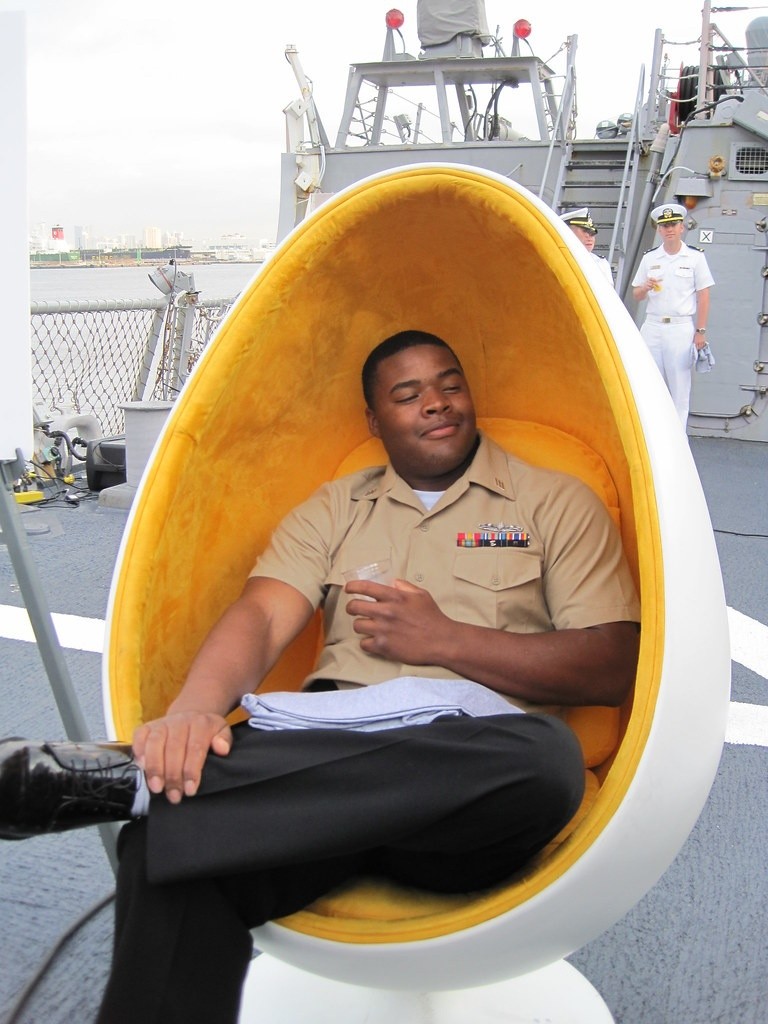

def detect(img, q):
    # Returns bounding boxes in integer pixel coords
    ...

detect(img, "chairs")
[102,161,732,1024]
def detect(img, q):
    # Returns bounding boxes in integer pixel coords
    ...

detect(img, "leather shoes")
[0,736,143,840]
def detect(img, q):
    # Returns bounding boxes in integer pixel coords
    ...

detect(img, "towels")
[688,339,715,374]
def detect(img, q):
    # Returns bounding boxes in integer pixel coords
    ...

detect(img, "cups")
[341,562,396,604]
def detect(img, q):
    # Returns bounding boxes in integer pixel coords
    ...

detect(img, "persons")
[631,204,715,432]
[0,331,640,1024]
[559,208,614,289]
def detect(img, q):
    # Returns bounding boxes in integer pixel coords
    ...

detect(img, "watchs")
[696,328,706,334]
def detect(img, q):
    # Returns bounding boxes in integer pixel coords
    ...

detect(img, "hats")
[558,207,592,229]
[650,204,688,224]
[587,213,598,233]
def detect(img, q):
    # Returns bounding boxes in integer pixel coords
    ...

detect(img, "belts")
[647,313,693,324]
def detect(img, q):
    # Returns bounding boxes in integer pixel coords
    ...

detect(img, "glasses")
[658,219,682,227]
